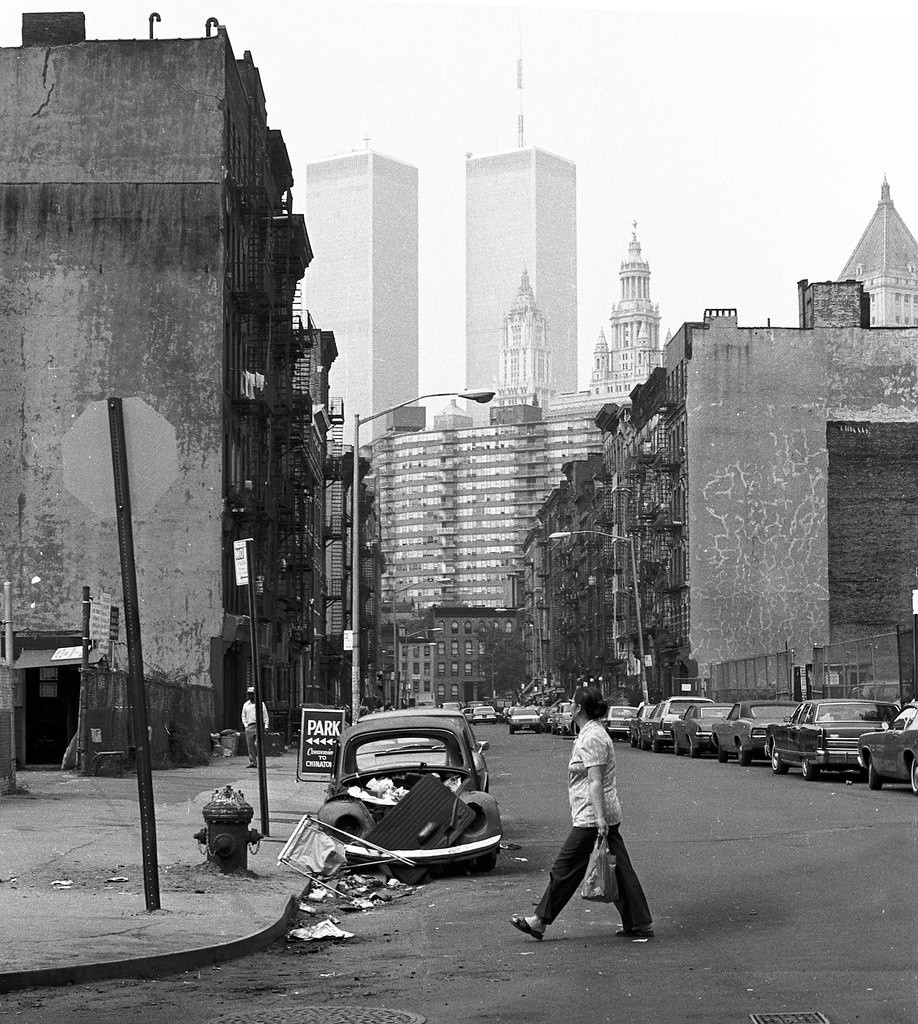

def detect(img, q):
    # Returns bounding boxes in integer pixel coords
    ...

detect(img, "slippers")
[615,926,654,938]
[509,916,544,940]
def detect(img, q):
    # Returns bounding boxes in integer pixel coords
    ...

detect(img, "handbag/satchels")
[581,834,618,903]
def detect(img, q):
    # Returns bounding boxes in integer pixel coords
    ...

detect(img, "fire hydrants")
[193,785,265,875]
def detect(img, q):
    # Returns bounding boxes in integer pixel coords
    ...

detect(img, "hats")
[246,687,255,693]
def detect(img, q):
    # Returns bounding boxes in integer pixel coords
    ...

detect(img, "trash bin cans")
[222,736,238,756]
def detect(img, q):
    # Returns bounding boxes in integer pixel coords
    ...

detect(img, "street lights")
[549,531,652,707]
[348,389,496,721]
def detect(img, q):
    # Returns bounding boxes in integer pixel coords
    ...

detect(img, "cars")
[508,707,544,733]
[669,702,734,758]
[353,709,491,795]
[857,701,918,796]
[326,715,504,880]
[471,706,496,725]
[628,704,658,751]
[440,699,513,721]
[764,699,902,783]
[599,706,639,738]
[710,700,802,766]
[525,700,577,737]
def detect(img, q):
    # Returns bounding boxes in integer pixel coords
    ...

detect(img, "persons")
[509,687,655,941]
[242,687,270,768]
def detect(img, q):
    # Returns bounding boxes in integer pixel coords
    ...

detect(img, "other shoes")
[246,764,257,768]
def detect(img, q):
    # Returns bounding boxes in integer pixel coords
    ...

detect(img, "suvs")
[641,696,715,753]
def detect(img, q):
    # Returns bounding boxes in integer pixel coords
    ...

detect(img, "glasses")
[568,699,577,705]
